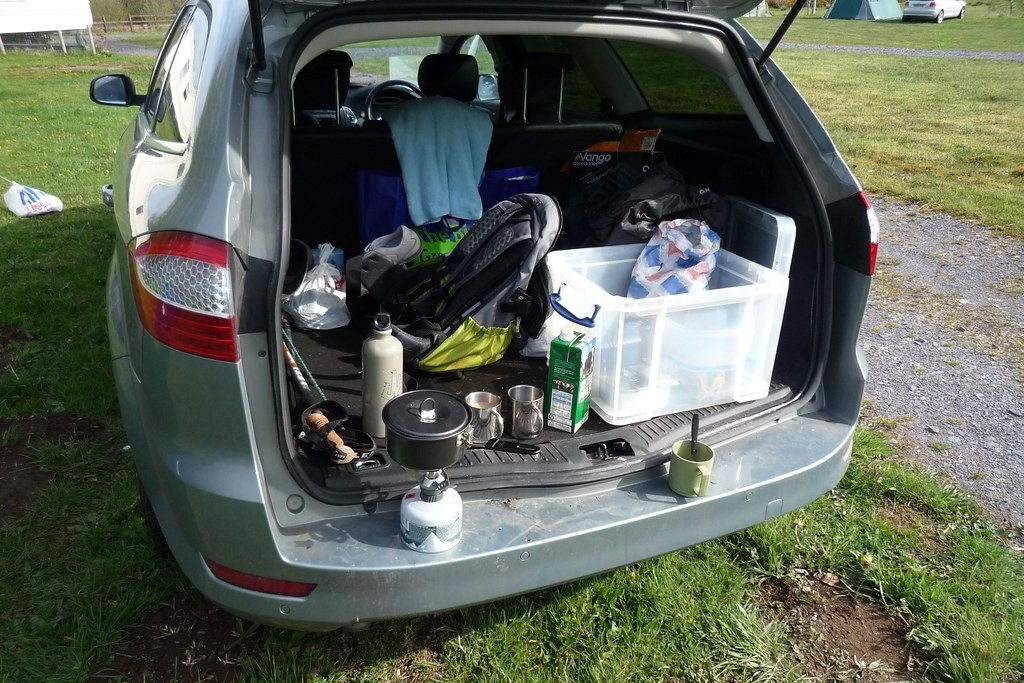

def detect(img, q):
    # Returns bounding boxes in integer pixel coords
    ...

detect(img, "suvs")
[89,0,881,628]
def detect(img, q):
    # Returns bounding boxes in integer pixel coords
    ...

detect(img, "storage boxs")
[546,243,789,425]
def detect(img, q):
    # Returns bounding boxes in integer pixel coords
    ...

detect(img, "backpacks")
[382,192,564,373]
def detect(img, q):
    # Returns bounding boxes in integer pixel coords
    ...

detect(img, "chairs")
[418,53,499,124]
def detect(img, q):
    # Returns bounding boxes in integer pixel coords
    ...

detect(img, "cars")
[902,0,965,24]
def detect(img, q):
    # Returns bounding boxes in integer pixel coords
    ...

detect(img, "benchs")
[290,50,622,253]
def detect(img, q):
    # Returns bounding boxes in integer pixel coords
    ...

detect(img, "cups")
[506,385,545,440]
[668,439,716,498]
[465,391,504,442]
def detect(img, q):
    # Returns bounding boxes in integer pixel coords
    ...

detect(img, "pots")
[380,390,541,470]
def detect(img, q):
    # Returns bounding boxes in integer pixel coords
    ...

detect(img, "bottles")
[362,313,403,438]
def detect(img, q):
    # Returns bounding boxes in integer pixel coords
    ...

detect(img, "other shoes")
[362,225,423,264]
